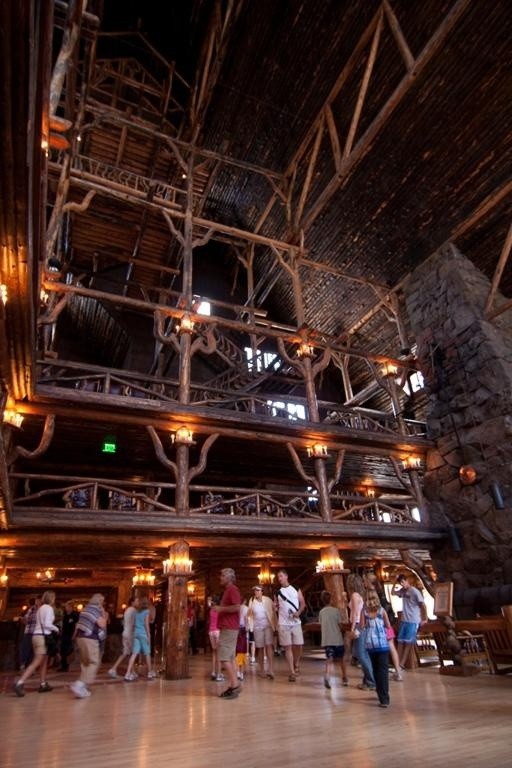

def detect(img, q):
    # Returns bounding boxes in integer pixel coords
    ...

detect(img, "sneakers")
[264,667,300,682]
[109,669,156,680]
[14,683,52,696]
[394,667,404,680]
[70,684,91,698]
[211,673,244,697]
[359,684,376,691]
[324,676,348,688]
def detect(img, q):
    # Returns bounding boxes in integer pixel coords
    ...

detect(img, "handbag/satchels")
[45,631,57,655]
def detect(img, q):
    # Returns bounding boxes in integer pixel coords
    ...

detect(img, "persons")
[319,590,349,689]
[213,568,242,700]
[14,590,59,697]
[273,568,306,682]
[245,585,277,679]
[237,592,268,680]
[392,574,428,669]
[348,572,408,684]
[187,595,224,683]
[148,596,166,660]
[108,596,139,679]
[68,593,107,698]
[57,601,80,674]
[124,594,153,680]
[360,588,393,706]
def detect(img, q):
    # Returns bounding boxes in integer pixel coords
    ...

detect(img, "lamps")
[162,558,194,574]
[307,444,327,457]
[171,429,192,443]
[296,345,313,357]
[2,409,24,428]
[132,575,155,587]
[456,367,507,510]
[258,573,275,585]
[315,558,345,571]
[401,457,421,468]
